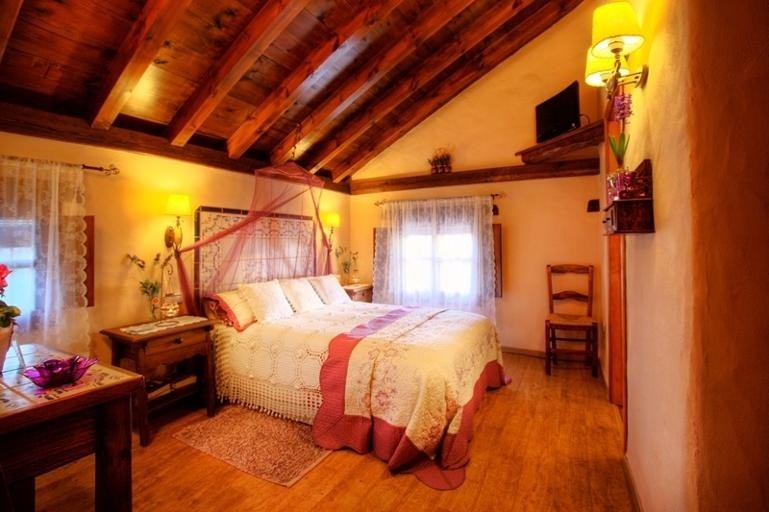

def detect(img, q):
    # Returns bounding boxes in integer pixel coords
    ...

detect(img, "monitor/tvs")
[535,80,581,144]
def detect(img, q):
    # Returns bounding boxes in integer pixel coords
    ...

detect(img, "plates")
[21,355,98,386]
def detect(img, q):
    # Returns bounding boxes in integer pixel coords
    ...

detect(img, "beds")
[211,300,512,491]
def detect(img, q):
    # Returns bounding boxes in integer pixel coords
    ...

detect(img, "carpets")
[172,409,334,488]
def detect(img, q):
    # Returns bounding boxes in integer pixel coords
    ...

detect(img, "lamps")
[165,195,191,248]
[327,213,339,248]
[584,2,649,99]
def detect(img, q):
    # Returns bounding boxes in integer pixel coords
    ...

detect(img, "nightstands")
[345,285,373,302]
[100,314,217,446]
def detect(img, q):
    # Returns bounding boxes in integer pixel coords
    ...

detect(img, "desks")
[0,343,144,511]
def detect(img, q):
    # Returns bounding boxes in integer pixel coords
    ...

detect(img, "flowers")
[127,253,161,321]
[427,149,450,164]
[0,264,21,327]
[606,94,632,169]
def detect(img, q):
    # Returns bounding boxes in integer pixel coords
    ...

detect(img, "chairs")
[544,264,598,378]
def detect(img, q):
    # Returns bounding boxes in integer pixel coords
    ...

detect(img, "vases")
[607,169,636,198]
[431,164,450,174]
[145,295,159,320]
[0,325,15,371]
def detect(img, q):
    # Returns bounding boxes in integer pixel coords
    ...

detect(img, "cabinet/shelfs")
[603,160,653,233]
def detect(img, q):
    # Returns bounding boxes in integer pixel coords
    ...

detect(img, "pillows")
[205,274,350,332]
